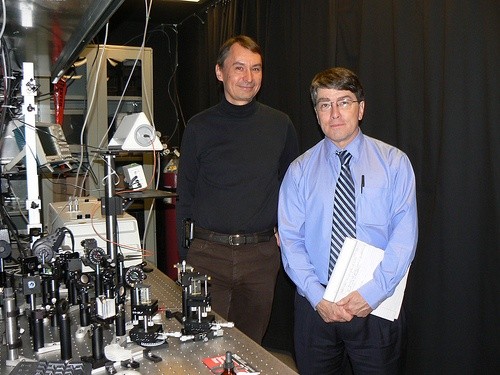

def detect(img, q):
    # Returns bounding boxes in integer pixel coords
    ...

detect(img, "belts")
[194,229,275,247]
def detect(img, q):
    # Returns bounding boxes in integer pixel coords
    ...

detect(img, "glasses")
[313,98,360,112]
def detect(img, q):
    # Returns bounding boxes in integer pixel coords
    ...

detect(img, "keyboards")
[7,359,92,375]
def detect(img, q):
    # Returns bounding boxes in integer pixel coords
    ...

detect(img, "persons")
[277,67,418,374]
[175,35,301,346]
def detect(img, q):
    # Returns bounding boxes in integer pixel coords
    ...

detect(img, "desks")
[0,260,300,375]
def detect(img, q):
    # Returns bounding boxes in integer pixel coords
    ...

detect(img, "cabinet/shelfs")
[38,44,157,267]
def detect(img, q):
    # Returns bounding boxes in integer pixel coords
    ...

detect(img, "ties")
[327,150,356,282]
